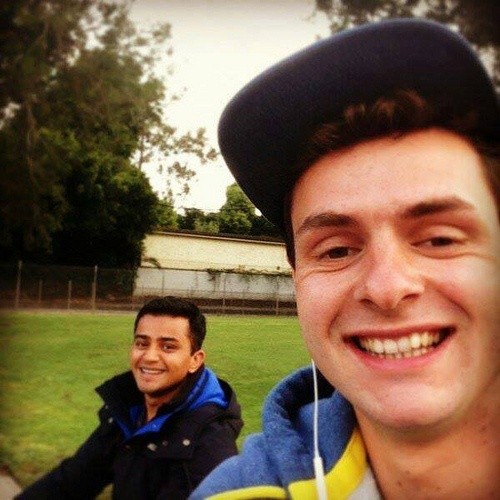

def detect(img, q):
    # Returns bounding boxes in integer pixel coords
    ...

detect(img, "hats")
[217,17,500,232]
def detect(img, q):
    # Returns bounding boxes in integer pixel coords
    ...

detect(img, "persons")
[186,19,499,500]
[11,294,246,500]
